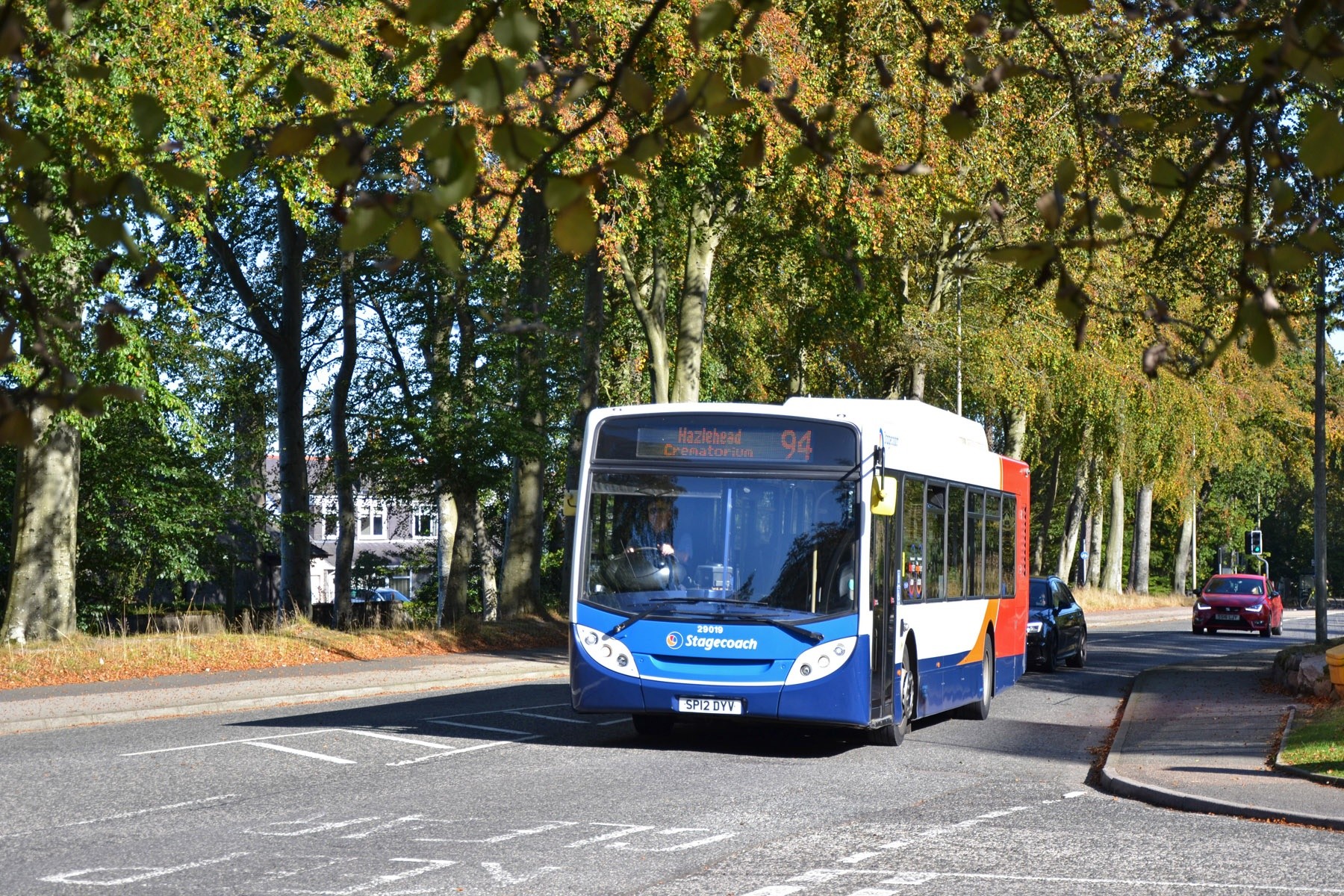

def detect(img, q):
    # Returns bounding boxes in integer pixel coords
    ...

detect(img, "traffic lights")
[1251,530,1262,555]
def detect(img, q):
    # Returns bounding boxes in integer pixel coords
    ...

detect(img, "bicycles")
[1299,586,1338,610]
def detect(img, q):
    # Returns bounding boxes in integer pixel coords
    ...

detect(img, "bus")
[566,398,1032,747]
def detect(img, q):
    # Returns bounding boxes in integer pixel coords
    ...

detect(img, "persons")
[1247,583,1260,594]
[1034,590,1049,606]
[623,497,692,591]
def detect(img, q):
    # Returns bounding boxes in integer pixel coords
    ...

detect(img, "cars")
[1026,575,1088,673]
[330,587,414,604]
[1191,573,1284,637]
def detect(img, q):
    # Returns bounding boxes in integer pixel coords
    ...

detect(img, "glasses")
[650,507,670,515]
